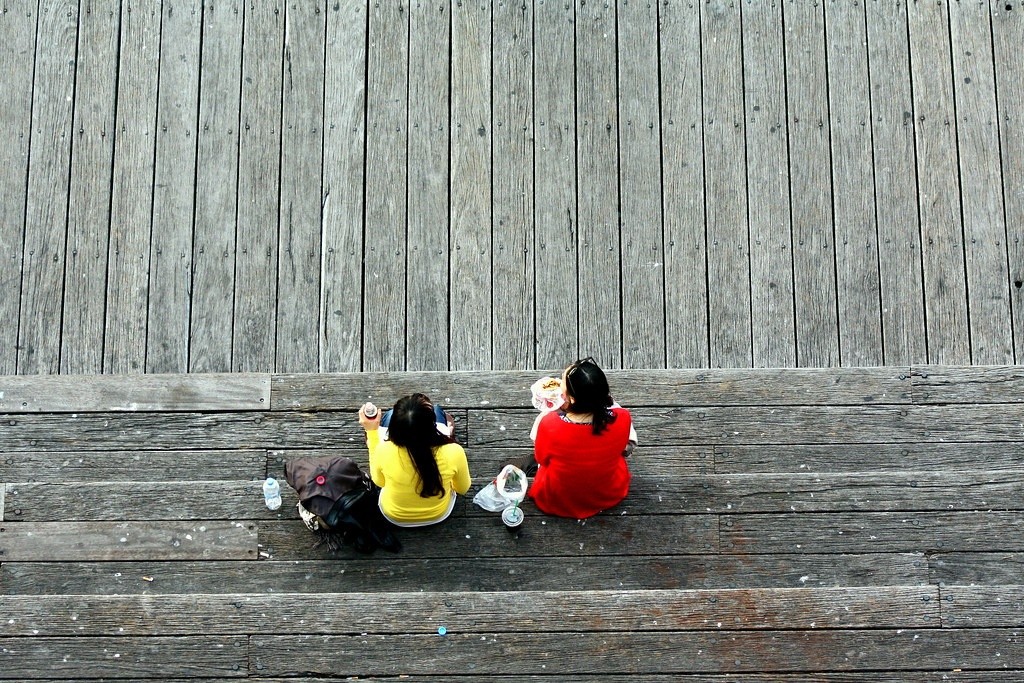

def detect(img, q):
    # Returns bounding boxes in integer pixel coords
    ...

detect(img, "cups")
[501,506,524,527]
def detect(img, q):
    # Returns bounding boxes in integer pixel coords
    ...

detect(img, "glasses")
[566,356,598,395]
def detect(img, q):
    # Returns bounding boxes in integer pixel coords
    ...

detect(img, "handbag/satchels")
[284,458,397,555]
[473,465,528,513]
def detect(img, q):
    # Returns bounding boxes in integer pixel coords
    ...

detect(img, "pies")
[543,381,560,390]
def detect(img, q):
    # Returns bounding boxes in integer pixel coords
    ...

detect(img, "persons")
[500,356,638,521]
[359,393,470,530]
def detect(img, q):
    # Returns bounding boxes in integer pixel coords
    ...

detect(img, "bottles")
[263,478,283,511]
[363,402,378,420]
